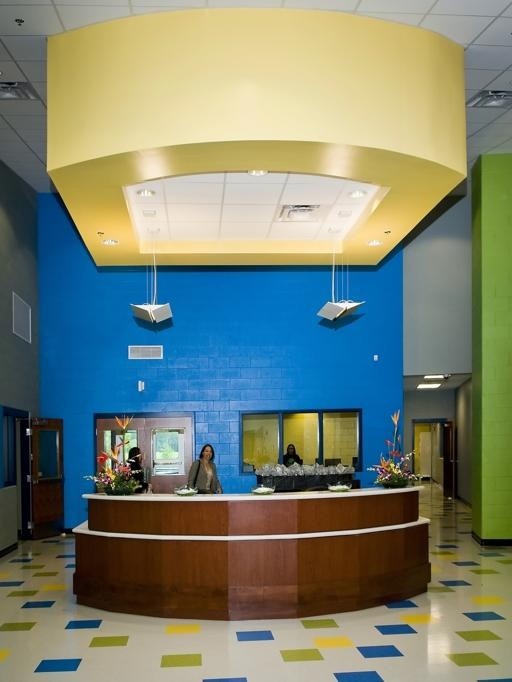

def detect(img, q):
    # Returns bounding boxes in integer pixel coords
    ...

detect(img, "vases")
[384,480,408,488]
[104,487,131,495]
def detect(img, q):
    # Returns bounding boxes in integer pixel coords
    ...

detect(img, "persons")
[283,443,303,468]
[188,444,223,494]
[126,447,153,493]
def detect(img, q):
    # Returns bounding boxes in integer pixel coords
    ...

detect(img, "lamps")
[130,210,172,323]
[316,224,365,321]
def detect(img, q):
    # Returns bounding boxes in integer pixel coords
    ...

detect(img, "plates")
[250,485,276,495]
[327,483,353,492]
[173,488,198,497]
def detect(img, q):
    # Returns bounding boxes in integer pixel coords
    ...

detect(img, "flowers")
[81,414,143,493]
[366,410,423,485]
